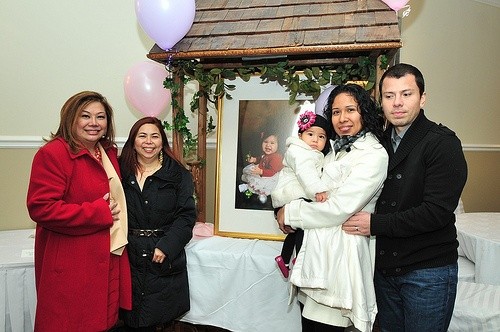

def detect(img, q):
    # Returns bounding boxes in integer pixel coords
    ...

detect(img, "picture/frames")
[213,70,338,242]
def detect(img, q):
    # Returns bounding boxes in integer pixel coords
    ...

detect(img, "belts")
[128,229,167,237]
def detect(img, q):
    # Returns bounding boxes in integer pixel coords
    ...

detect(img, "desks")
[0,210,500,332]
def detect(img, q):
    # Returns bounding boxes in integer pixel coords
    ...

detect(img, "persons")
[271,110,334,278]
[276,84,389,332]
[117,117,197,332]
[26,91,132,332]
[341,63,468,332]
[239,131,284,203]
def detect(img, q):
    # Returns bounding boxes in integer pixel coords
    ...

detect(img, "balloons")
[125,61,171,118]
[382,0,411,13]
[135,0,196,52]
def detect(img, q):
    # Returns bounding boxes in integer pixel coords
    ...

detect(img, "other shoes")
[275,256,291,278]
[292,258,296,265]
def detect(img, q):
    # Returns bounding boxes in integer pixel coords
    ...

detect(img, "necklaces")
[141,163,158,172]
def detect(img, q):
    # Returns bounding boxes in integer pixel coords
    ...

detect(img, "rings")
[355,226,358,231]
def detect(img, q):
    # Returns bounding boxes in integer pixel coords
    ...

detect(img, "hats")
[298,111,328,133]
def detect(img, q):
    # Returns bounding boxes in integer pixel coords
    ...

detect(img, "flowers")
[297,110,316,130]
